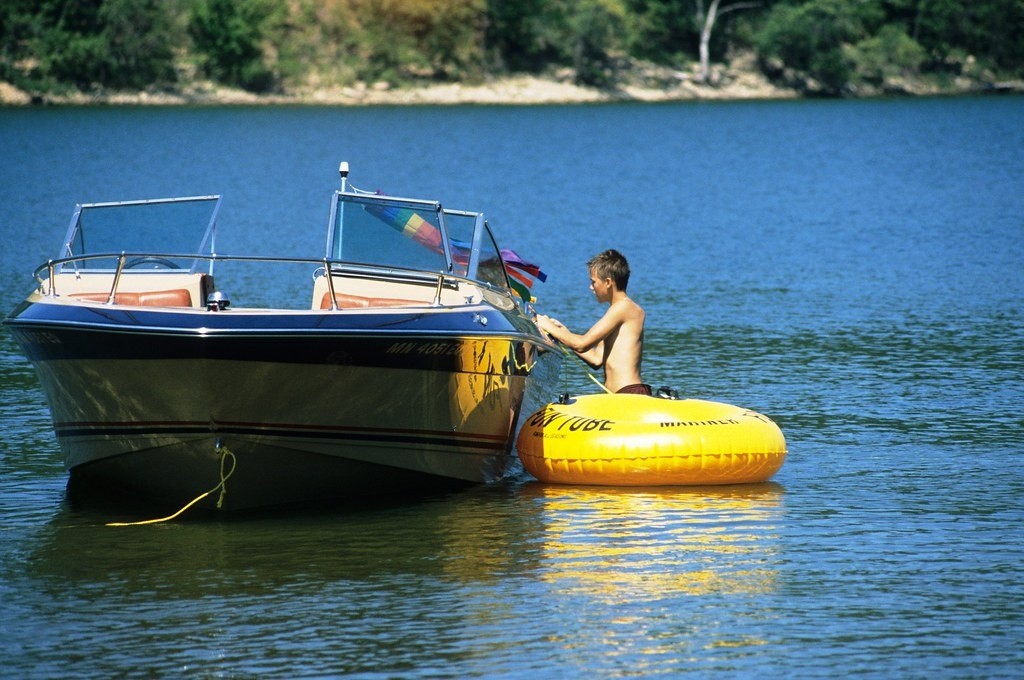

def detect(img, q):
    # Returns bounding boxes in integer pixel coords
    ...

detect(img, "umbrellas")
[360,191,613,395]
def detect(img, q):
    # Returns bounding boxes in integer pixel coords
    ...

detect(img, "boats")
[0,161,568,513]
[517,383,787,487]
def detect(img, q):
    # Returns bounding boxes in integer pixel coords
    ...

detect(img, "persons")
[537,249,652,395]
